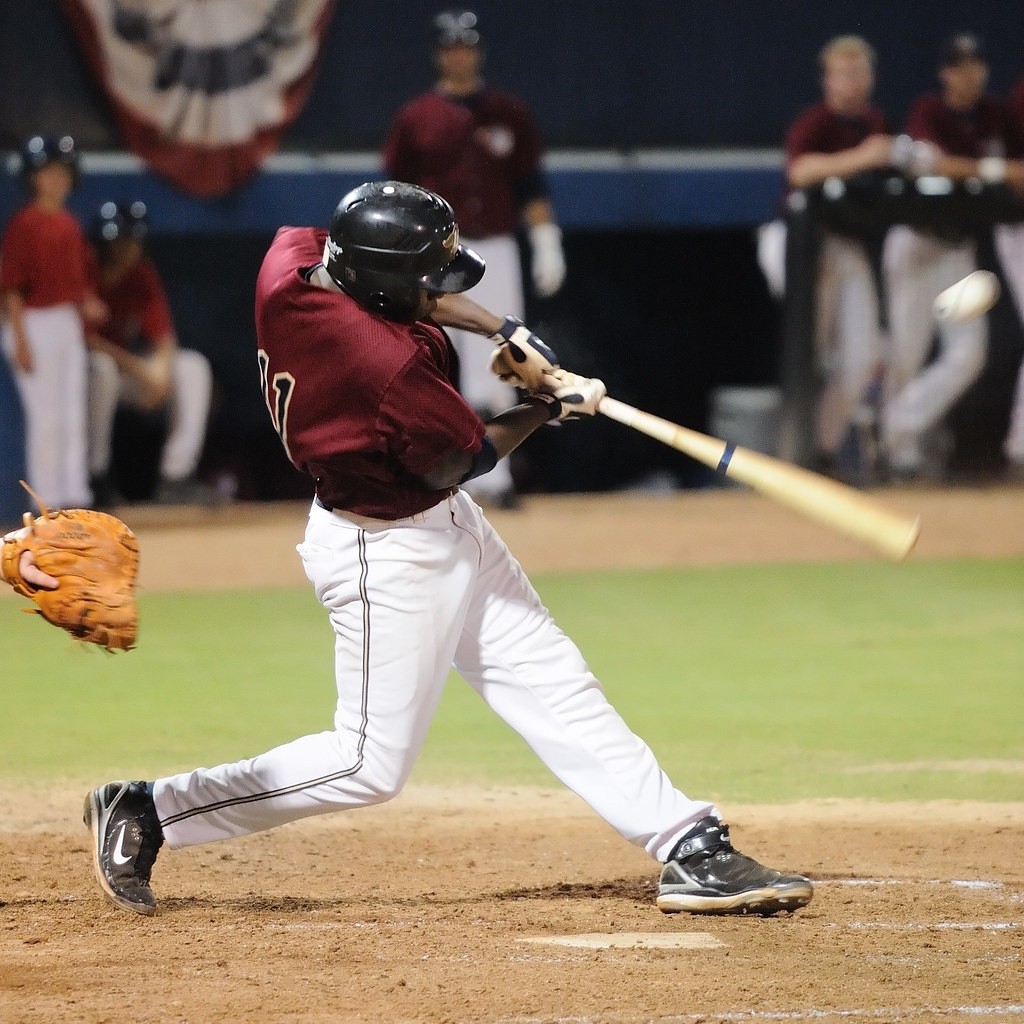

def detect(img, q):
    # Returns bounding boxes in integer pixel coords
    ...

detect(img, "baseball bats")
[496,354,924,565]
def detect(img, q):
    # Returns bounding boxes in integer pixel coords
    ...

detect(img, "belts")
[316,485,460,522]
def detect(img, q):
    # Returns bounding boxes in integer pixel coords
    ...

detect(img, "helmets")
[322,180,486,319]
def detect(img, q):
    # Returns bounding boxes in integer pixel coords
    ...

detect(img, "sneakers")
[82,779,164,917]
[654,814,814,912]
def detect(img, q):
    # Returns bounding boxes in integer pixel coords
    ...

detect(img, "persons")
[0,11,1024,516]
[0,481,140,653]
[78,181,811,920]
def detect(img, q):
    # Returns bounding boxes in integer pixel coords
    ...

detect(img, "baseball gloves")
[1,505,142,652]
